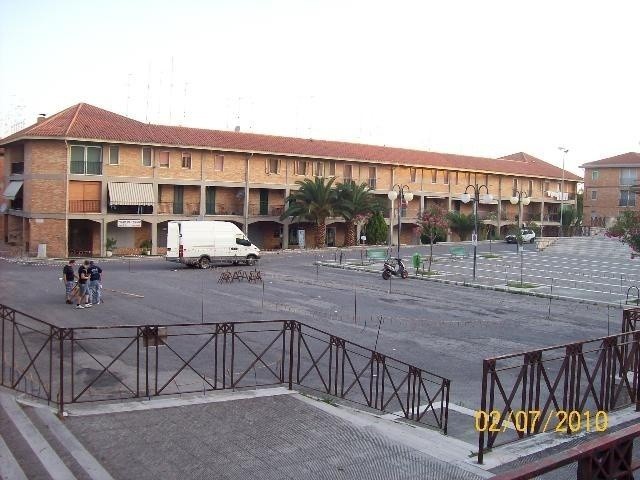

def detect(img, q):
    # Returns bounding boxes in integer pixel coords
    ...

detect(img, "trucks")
[165,219,263,270]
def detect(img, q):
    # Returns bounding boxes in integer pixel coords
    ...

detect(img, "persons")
[76,260,91,309]
[63,259,79,304]
[87,261,103,306]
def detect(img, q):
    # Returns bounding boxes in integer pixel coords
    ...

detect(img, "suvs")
[506,229,538,245]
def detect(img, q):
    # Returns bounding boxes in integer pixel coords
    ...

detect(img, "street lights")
[557,145,571,232]
[461,181,495,281]
[511,188,534,287]
[388,182,415,261]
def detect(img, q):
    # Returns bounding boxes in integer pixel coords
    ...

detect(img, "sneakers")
[66,299,92,309]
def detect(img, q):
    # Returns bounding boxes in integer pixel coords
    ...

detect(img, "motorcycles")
[382,257,411,281]
